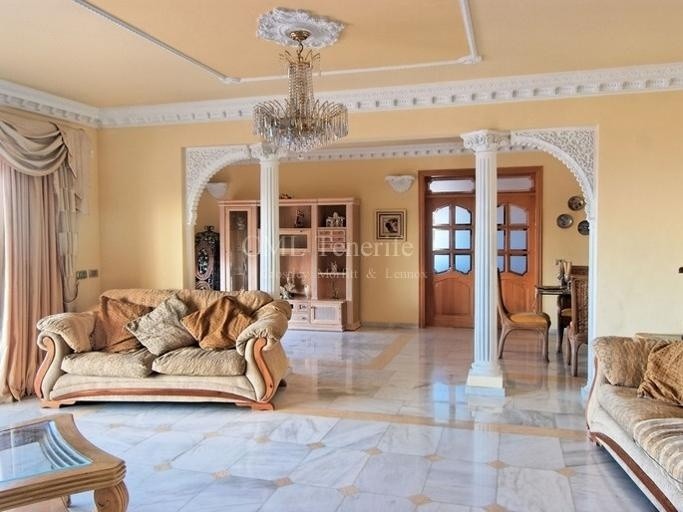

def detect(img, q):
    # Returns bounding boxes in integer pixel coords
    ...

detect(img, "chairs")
[493,264,551,363]
[563,272,592,376]
[554,260,594,356]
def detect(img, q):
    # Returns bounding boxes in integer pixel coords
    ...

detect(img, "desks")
[0,408,132,512]
[531,282,574,299]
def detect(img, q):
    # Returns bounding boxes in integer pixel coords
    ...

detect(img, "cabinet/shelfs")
[250,197,318,300]
[310,301,348,333]
[276,299,313,330]
[216,198,256,290]
[312,195,363,331]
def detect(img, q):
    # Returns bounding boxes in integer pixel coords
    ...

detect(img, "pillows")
[638,337,682,406]
[125,293,198,356]
[96,292,154,353]
[592,333,658,388]
[179,294,252,352]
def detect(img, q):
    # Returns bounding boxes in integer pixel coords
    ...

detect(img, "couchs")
[34,285,295,413]
[580,326,683,512]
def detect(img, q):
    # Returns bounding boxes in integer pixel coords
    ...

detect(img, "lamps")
[383,175,415,194]
[204,181,227,201]
[250,28,348,155]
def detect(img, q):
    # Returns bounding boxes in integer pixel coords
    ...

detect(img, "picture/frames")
[372,208,407,242]
